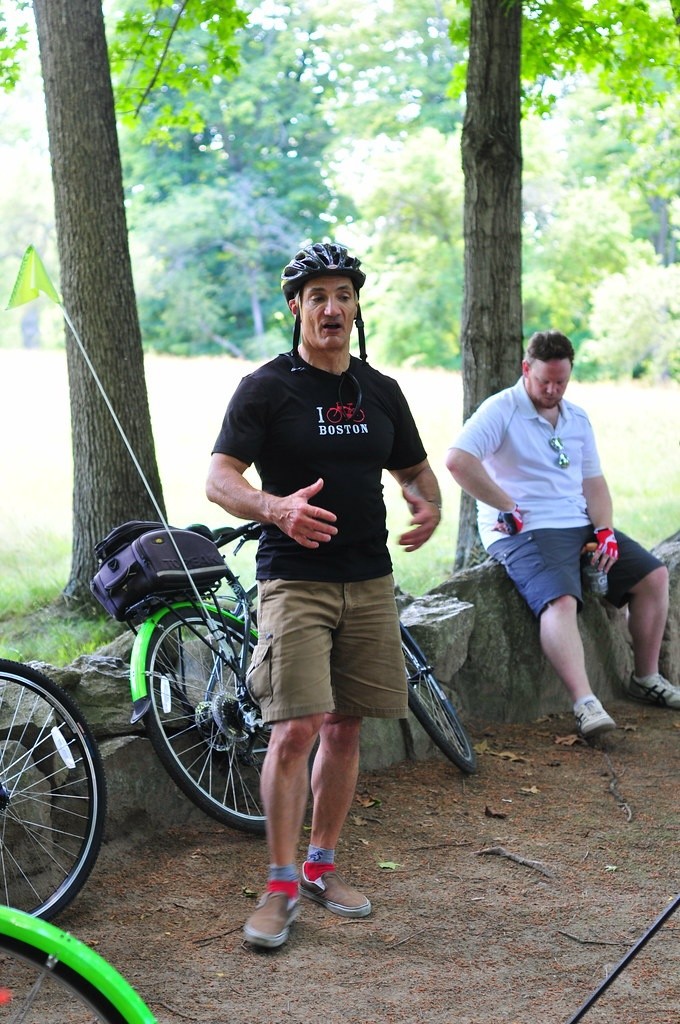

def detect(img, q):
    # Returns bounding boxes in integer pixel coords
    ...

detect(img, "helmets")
[281,242,367,309]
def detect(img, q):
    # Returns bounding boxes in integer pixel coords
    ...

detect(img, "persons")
[205,243,442,946]
[446,331,680,737]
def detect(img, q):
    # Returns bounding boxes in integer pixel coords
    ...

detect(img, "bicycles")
[120,519,479,837]
[0,659,109,924]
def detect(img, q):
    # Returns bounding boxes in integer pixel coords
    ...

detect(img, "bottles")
[583,543,608,596]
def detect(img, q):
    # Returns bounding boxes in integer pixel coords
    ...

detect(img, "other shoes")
[299,861,372,918]
[244,893,301,948]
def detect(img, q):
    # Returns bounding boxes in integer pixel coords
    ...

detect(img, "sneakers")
[575,699,615,736]
[627,670,679,709]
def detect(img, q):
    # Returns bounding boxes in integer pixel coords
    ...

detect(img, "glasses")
[550,436,570,469]
[338,370,362,423]
